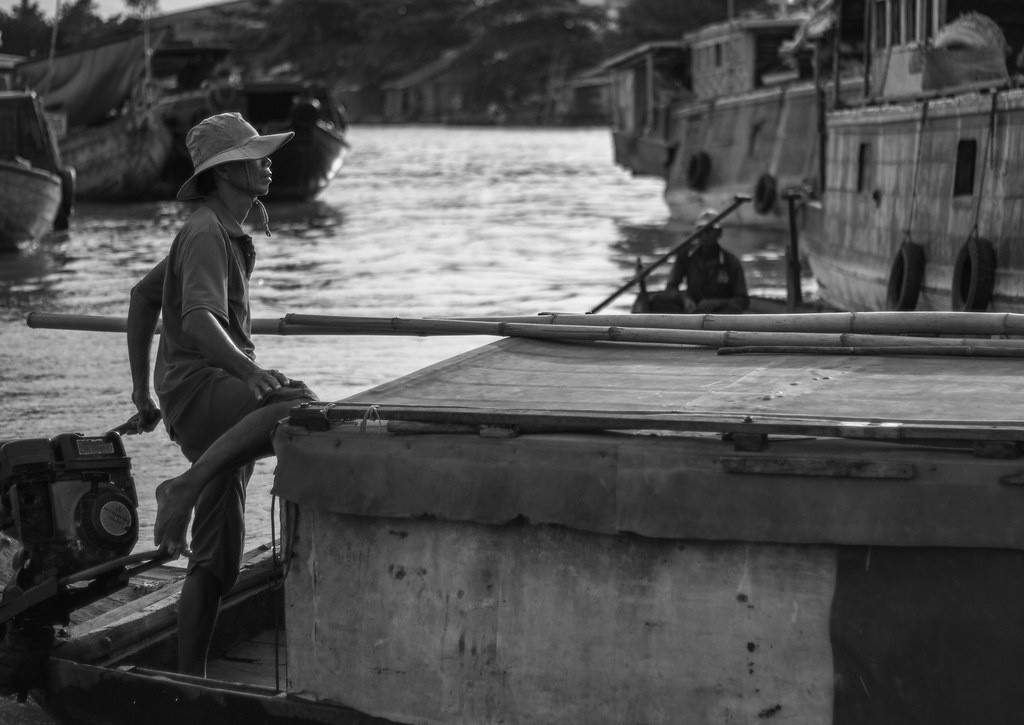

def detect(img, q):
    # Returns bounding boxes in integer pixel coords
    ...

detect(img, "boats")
[0,300,1023,724]
[591,0,1024,311]
[0,94,82,254]
[42,6,357,204]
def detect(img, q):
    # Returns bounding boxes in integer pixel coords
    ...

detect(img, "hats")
[695,211,725,230]
[175,112,296,201]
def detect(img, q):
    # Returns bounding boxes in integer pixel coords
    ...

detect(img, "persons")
[664,209,750,314]
[119,114,316,677]
